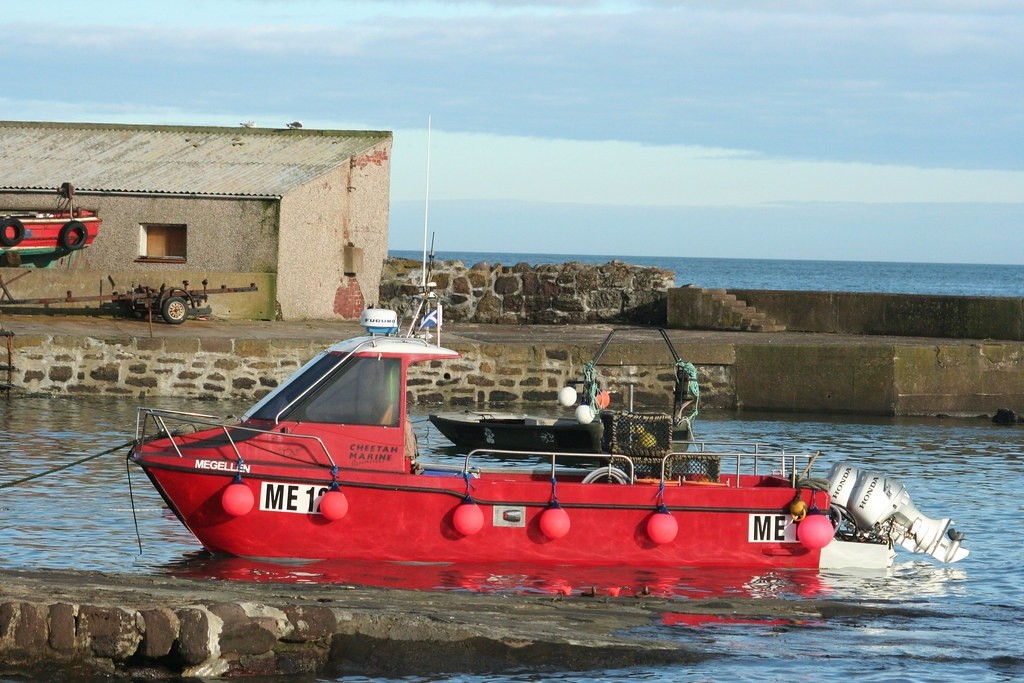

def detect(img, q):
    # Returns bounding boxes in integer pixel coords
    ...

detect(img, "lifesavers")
[58,220,88,251]
[0,218,25,247]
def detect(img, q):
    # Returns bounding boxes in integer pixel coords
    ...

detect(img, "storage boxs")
[600,410,719,484]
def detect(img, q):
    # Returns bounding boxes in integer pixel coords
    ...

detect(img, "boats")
[429,326,702,467]
[127,114,970,603]
[4,183,102,269]
